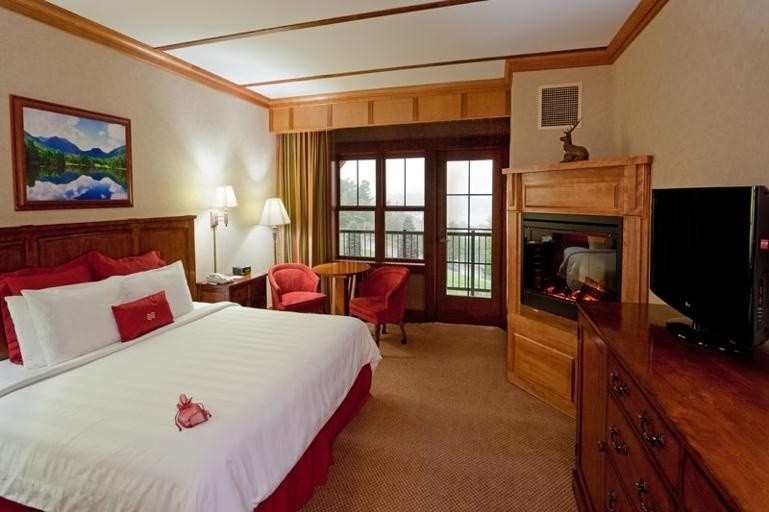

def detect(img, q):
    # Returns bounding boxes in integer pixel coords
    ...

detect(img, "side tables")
[310,262,370,318]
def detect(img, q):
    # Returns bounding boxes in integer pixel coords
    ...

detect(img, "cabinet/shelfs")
[573,297,768,512]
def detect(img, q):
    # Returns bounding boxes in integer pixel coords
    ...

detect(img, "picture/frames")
[8,95,134,212]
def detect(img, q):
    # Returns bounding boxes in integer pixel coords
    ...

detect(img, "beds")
[0,215,372,512]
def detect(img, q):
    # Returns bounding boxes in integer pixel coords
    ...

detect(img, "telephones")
[206,273,234,285]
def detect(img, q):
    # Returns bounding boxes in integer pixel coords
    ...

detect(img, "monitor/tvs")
[648,185,769,351]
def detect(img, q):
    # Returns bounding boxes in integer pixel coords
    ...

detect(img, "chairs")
[267,263,327,314]
[348,263,410,346]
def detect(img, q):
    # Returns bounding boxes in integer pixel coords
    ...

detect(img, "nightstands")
[196,271,268,308]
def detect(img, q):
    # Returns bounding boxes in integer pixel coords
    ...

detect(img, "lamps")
[258,199,292,265]
[210,185,238,228]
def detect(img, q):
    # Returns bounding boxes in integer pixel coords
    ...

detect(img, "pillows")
[1,248,194,369]
